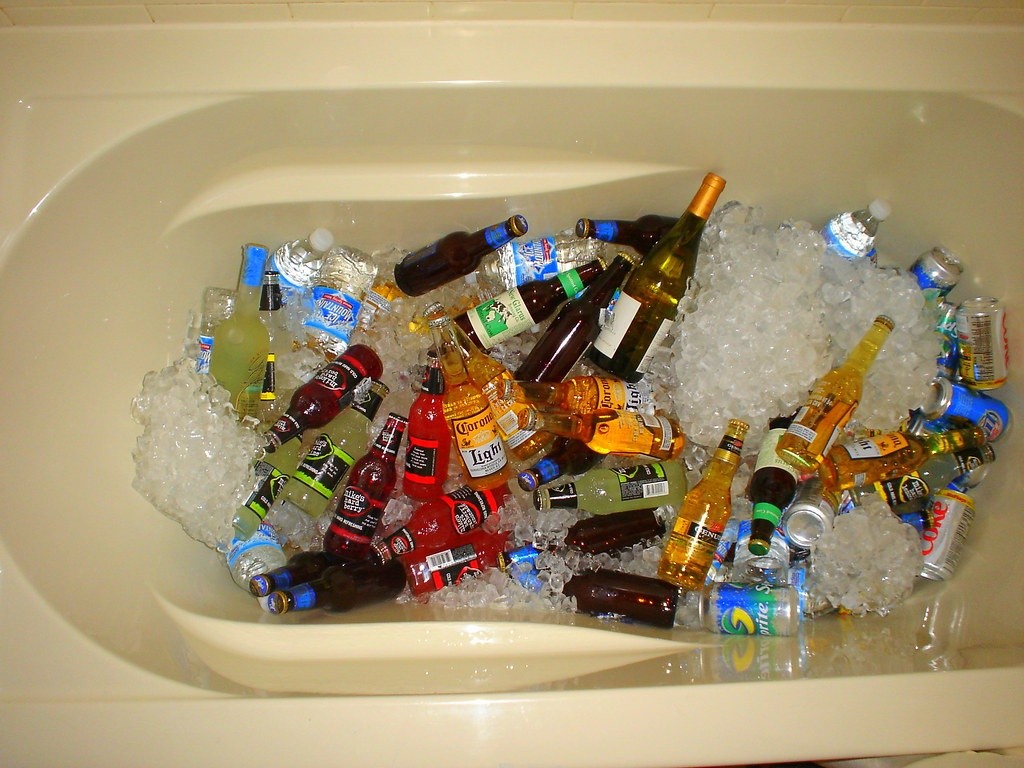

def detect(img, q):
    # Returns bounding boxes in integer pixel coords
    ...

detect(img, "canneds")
[782,476,843,549]
[900,410,990,581]
[910,244,1011,441]
[697,580,798,635]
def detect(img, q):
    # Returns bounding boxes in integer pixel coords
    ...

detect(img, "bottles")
[581,172,725,382]
[575,214,682,257]
[748,416,799,556]
[224,301,599,614]
[819,427,984,489]
[394,214,528,297]
[775,313,895,473]
[513,251,635,383]
[305,245,378,360]
[817,200,891,261]
[510,568,679,628]
[657,419,750,591]
[496,377,656,413]
[793,509,934,618]
[532,459,688,515]
[259,271,289,419]
[268,227,334,287]
[496,507,666,570]
[209,243,269,420]
[517,408,684,460]
[500,228,608,283]
[849,443,996,508]
[453,257,607,351]
